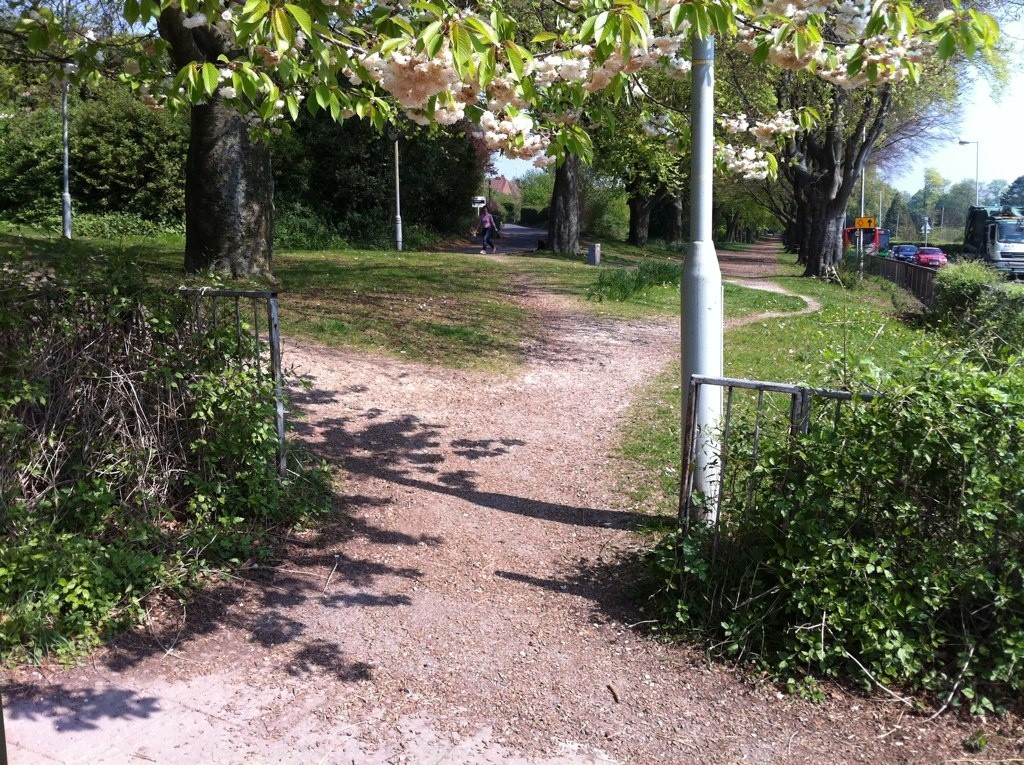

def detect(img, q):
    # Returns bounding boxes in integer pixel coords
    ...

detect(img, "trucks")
[963,205,1024,282]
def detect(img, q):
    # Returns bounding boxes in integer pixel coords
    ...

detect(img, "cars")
[890,244,919,263]
[912,246,948,270]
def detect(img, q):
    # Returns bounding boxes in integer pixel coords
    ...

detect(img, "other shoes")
[492,247,496,253]
[480,250,487,254]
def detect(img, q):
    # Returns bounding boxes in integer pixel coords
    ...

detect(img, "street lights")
[958,140,979,205]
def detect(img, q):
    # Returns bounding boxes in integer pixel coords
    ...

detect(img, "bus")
[842,227,879,257]
[878,228,891,258]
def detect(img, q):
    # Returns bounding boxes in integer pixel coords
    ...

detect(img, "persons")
[473,206,498,254]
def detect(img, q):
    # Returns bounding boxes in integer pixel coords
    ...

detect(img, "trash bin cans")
[589,244,601,267]
